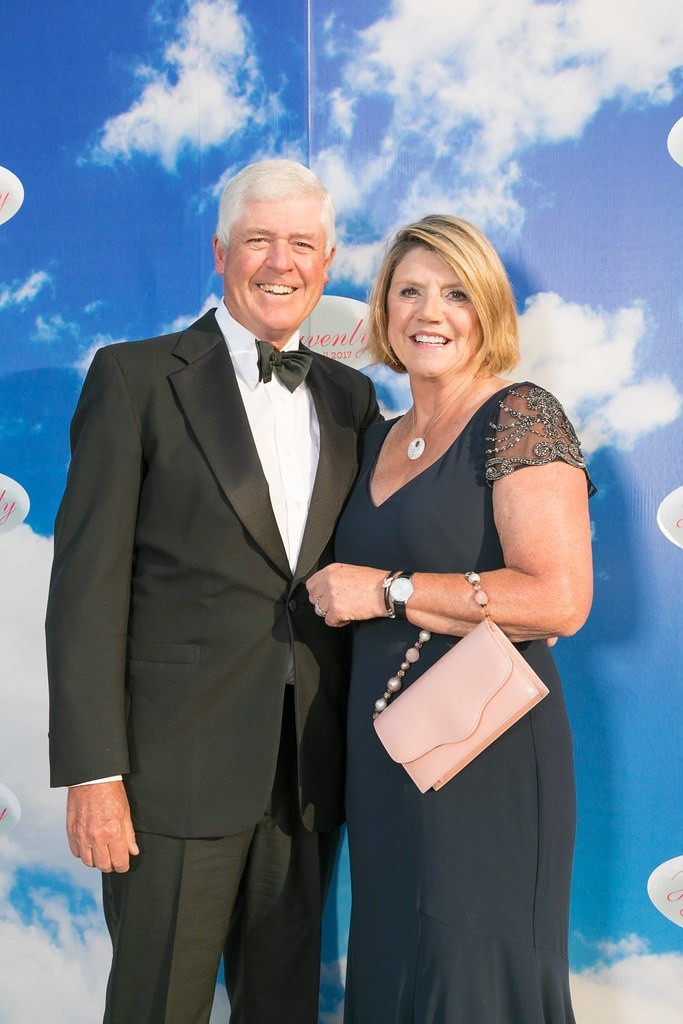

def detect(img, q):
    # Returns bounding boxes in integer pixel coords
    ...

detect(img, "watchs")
[389,569,414,619]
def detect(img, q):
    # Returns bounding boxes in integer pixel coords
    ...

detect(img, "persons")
[305,216,597,1023]
[46,159,386,1024]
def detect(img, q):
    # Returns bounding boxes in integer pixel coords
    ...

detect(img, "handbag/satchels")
[373,571,549,793]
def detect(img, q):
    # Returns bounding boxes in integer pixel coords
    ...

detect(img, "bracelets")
[381,568,403,620]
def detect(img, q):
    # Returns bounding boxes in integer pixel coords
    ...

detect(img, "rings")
[314,597,326,617]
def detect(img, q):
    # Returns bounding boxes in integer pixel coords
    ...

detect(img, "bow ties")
[255,338,313,394]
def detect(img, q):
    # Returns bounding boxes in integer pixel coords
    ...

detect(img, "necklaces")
[407,375,478,460]
